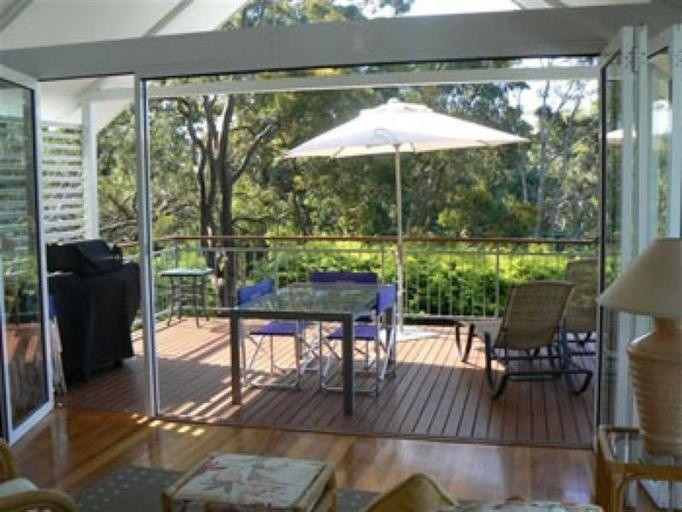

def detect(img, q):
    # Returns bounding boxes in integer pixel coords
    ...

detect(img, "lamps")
[597,238,682,456]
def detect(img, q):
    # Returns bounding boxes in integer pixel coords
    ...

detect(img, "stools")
[156,450,337,511]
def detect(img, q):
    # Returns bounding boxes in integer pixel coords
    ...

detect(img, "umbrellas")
[281,97,532,334]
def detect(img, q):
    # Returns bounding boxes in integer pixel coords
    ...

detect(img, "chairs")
[0,442,76,512]
[237,269,398,399]
[453,257,601,399]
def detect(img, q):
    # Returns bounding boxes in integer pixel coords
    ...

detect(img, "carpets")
[73,462,489,512]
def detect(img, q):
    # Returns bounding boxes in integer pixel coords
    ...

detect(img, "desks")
[217,281,384,418]
[159,267,214,328]
[594,421,682,512]
